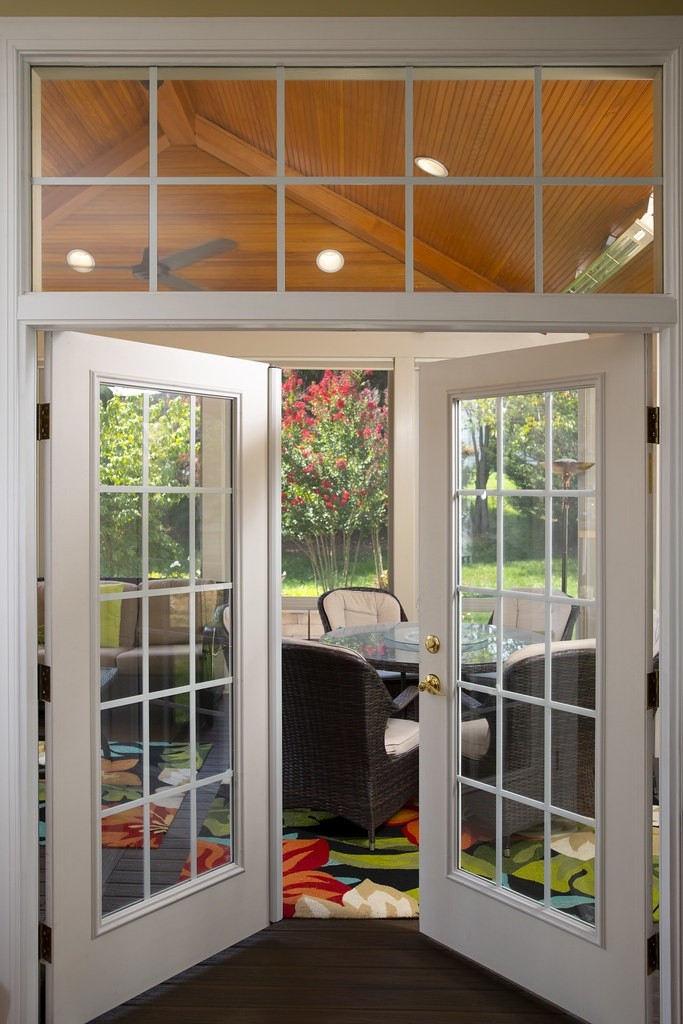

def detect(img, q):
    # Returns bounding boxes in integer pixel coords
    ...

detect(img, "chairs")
[214,586,659,859]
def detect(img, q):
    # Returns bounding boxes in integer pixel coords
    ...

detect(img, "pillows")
[98,583,122,648]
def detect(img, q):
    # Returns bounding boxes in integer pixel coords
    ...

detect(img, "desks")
[319,622,546,723]
[99,665,120,758]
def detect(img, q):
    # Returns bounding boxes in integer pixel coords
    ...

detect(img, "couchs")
[38,578,229,731]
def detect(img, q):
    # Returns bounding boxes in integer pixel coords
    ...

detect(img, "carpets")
[180,777,660,926]
[39,737,212,849]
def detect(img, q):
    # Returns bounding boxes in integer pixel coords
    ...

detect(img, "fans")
[45,235,237,292]
